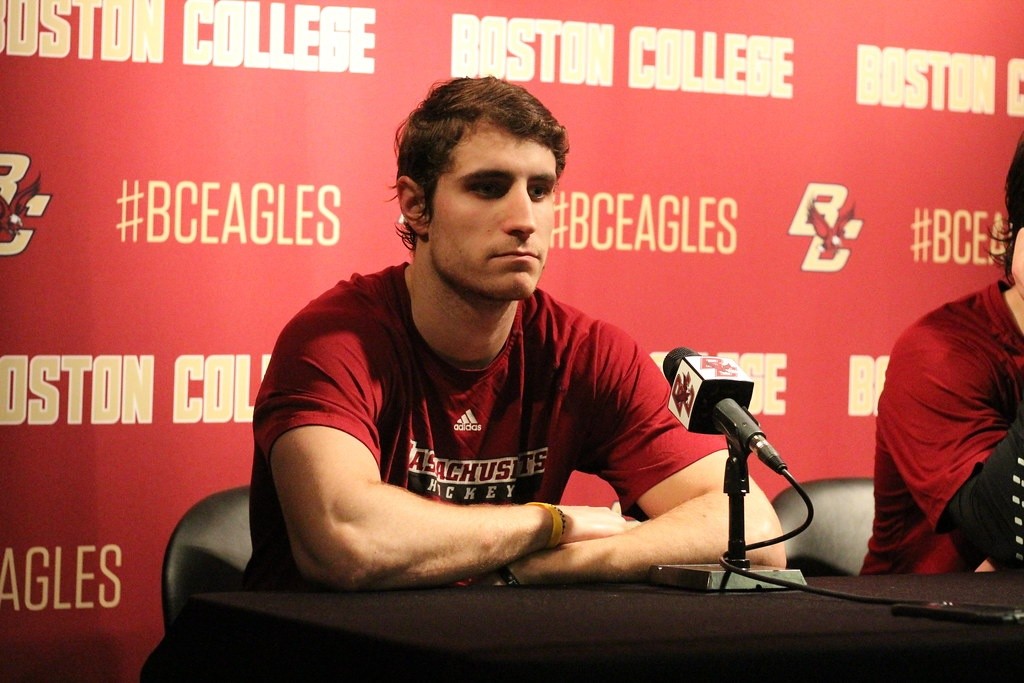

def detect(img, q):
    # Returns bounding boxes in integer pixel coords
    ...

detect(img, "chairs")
[772,477,875,578]
[162,484,254,635]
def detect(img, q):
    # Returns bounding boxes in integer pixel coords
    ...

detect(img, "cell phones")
[892,601,1024,624]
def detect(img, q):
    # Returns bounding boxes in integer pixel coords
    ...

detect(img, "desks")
[137,571,1022,682]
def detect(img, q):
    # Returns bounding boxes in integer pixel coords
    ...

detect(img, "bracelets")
[528,502,567,549]
[498,566,520,585]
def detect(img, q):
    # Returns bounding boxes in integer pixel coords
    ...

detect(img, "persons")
[242,79,788,591]
[860,134,1024,577]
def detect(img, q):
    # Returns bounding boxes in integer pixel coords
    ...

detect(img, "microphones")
[663,347,788,474]
